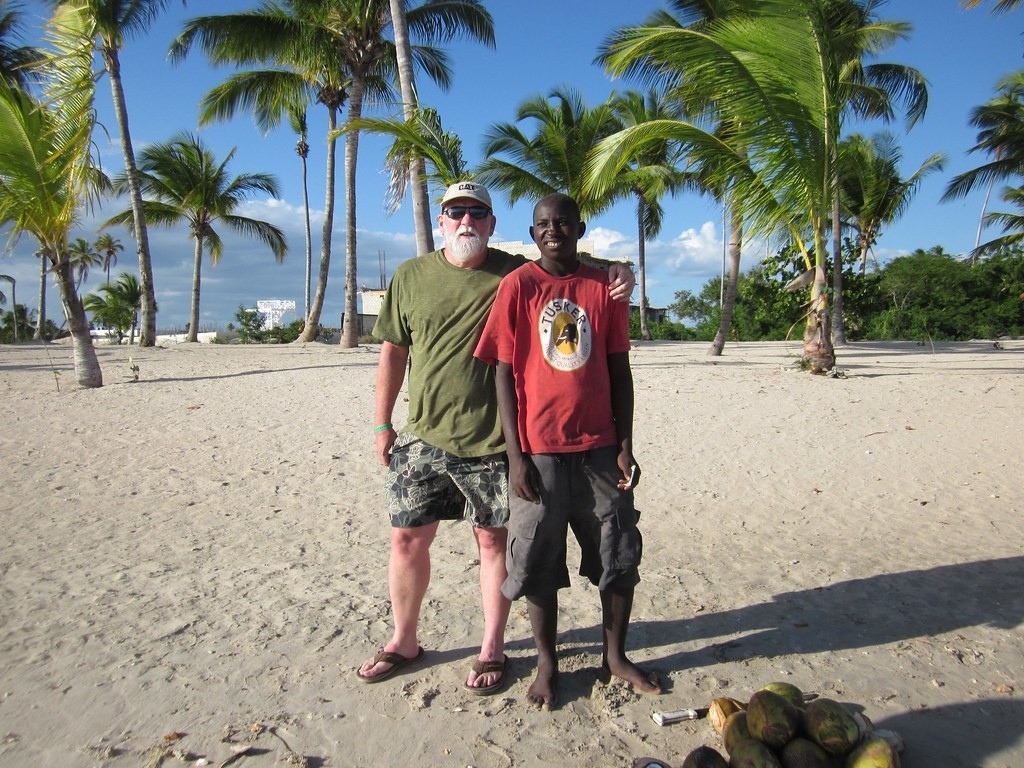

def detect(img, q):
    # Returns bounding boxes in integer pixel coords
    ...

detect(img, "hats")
[440,181,492,210]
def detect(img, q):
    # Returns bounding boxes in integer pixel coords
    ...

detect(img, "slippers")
[355,645,425,682]
[463,653,509,696]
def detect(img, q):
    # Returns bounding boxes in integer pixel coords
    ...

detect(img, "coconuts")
[685,681,900,768]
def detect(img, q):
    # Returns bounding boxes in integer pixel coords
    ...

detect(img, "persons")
[478,193,670,714]
[352,188,637,694]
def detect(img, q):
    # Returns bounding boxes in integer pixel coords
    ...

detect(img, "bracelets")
[369,422,394,434]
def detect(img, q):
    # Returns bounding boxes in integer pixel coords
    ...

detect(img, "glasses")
[442,205,493,220]
